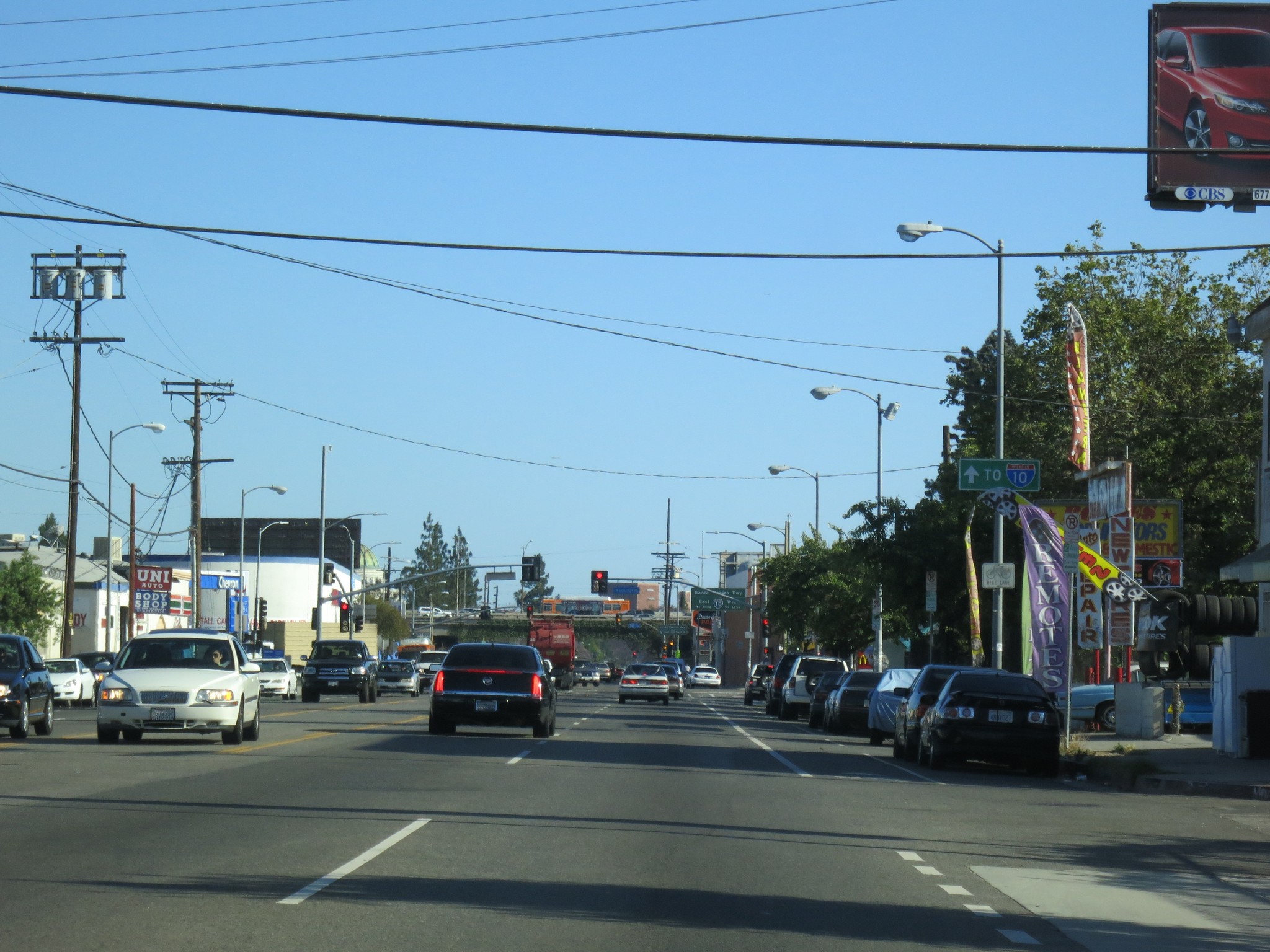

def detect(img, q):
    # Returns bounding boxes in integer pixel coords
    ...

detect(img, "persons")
[208,645,224,667]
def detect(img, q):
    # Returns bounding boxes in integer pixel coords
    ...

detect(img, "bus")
[542,596,630,616]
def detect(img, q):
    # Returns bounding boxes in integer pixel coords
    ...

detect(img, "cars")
[745,652,1166,780]
[1154,25,1270,165]
[0,635,120,740]
[525,556,728,706]
[452,608,493,617]
[248,638,448,703]
[428,643,559,739]
[91,627,262,744]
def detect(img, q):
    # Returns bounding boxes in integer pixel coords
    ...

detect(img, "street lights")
[895,223,1011,674]
[747,521,788,656]
[812,384,906,673]
[105,424,166,655]
[237,486,290,657]
[428,591,449,640]
[768,464,821,548]
[707,531,767,644]
[314,445,390,646]
[338,524,450,640]
[521,540,534,613]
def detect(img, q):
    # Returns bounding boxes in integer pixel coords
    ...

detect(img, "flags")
[1017,504,1070,693]
[979,487,1150,604]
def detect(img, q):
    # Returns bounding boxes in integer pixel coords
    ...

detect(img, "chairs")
[6,651,18,666]
[382,665,392,672]
[65,665,73,673]
[146,643,171,667]
[201,642,230,671]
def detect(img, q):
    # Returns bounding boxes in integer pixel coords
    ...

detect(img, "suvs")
[416,607,453,618]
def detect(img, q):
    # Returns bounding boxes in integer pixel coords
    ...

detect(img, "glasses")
[209,653,222,658]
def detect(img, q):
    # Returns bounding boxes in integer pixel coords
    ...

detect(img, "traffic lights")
[763,647,771,654]
[260,598,268,618]
[760,615,771,639]
[591,571,608,594]
